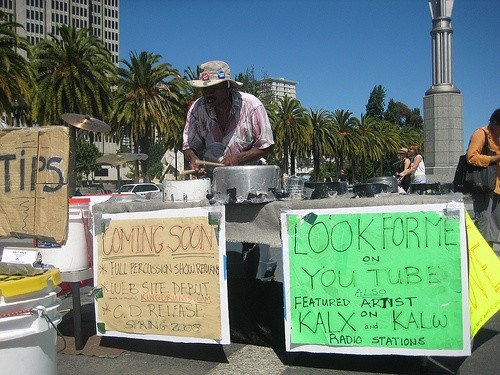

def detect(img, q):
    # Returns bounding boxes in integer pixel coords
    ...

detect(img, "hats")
[187,61,244,89]
[398,147,408,154]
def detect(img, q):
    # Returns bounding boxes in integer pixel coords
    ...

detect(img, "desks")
[58,269,93,351]
[227,193,466,227]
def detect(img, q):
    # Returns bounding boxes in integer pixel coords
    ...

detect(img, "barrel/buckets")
[0,268,67,375]
[35,193,115,271]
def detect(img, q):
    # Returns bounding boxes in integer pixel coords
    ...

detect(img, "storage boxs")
[66,197,90,272]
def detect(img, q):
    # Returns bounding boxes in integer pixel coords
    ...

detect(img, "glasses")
[409,148,413,150]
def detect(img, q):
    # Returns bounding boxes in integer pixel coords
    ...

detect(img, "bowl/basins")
[203,142,232,178]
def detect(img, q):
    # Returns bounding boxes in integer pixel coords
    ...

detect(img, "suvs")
[111,183,162,199]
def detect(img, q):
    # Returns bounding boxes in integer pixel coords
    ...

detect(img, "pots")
[212,164,441,204]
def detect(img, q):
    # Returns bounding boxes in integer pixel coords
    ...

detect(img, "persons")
[467,109,500,249]
[183,60,275,179]
[398,148,411,195]
[324,172,333,182]
[338,170,349,185]
[399,144,426,194]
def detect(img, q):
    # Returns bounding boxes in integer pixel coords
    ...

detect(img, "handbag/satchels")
[452,128,497,194]
